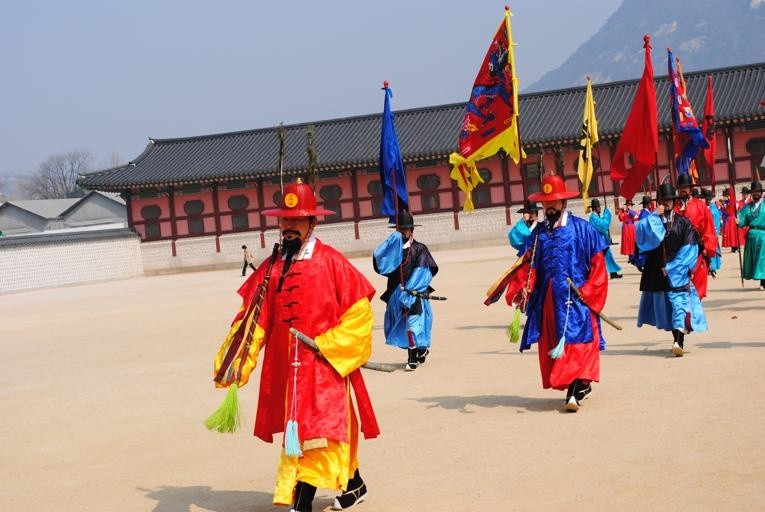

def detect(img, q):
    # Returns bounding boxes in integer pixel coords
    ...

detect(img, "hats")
[639,196,653,205]
[720,188,730,196]
[528,170,581,203]
[517,199,543,212]
[587,198,602,208]
[739,181,765,193]
[651,171,715,201]
[261,178,337,218]
[388,209,421,228]
[623,199,635,205]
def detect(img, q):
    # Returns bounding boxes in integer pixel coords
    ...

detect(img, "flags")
[607,42,659,199]
[574,80,601,211]
[665,48,718,184]
[377,86,408,223]
[448,13,528,212]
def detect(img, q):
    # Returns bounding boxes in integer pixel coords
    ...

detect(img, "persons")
[635,185,709,356]
[618,196,653,273]
[484,175,610,415]
[374,211,438,371]
[692,182,764,290]
[509,199,541,251]
[240,244,256,278]
[586,199,623,280]
[673,173,719,300]
[206,174,382,511]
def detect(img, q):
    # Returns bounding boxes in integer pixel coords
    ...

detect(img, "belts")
[750,226,765,230]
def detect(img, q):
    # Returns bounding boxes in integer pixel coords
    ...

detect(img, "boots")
[671,329,684,357]
[610,272,623,278]
[565,378,591,410]
[289,481,317,512]
[759,279,765,290]
[404,346,429,370]
[334,468,366,510]
